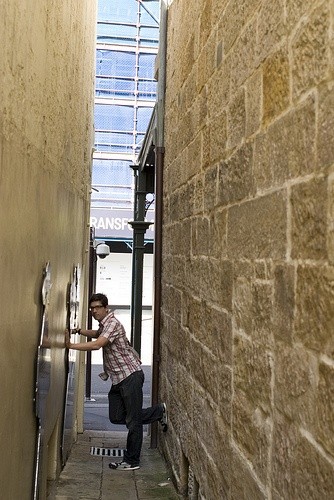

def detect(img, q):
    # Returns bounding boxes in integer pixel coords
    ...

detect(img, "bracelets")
[74,329,77,334]
[78,329,82,335]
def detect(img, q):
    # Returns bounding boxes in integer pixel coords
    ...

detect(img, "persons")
[65,293,168,470]
[40,313,78,414]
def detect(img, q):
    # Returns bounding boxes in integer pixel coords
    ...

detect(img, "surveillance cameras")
[96,244,110,259]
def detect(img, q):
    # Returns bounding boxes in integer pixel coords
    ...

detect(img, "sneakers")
[109,459,140,471]
[160,402,167,433]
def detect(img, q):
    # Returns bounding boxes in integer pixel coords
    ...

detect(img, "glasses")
[89,305,104,311]
